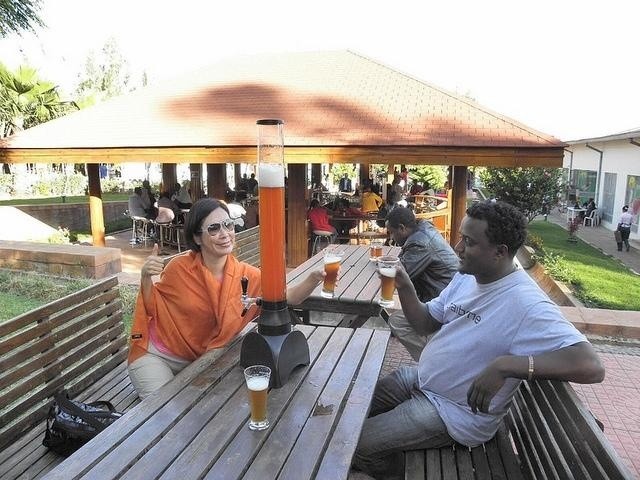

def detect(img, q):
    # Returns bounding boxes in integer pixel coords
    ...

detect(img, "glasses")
[195,217,236,238]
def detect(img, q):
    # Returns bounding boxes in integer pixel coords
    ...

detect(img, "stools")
[123,207,331,258]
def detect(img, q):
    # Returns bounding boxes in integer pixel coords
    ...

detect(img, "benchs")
[162,225,295,275]
[404,380,638,480]
[0,277,142,480]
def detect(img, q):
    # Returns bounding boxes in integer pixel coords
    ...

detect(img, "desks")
[39,320,392,480]
[286,243,402,328]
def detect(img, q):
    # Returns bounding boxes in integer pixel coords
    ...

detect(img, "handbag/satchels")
[43,399,125,458]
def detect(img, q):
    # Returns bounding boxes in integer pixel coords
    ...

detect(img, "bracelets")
[528,354,534,381]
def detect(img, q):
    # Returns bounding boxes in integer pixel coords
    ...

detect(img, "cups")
[370,239,383,262]
[244,364,272,434]
[320,249,342,297]
[377,256,401,303]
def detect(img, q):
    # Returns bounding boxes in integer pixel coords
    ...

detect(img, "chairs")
[559,200,600,228]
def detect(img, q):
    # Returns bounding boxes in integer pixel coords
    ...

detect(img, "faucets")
[239,276,258,316]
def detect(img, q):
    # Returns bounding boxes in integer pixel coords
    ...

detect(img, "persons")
[358,188,383,213]
[128,171,258,227]
[307,199,338,244]
[383,206,461,362]
[351,197,605,478]
[616,205,632,252]
[324,164,437,206]
[125,197,343,403]
[284,177,289,208]
